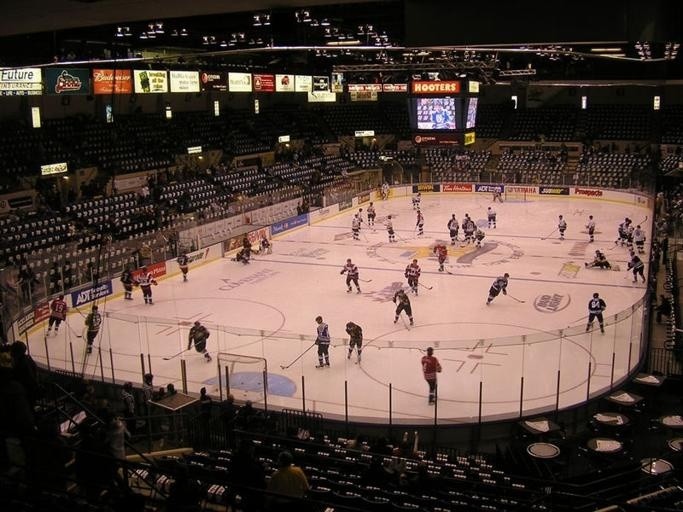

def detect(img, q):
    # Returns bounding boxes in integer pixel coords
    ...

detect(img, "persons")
[467,101,475,127]
[0,132,682,512]
[431,103,450,129]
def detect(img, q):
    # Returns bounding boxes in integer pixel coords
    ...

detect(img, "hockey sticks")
[507,294,525,303]
[457,235,474,247]
[409,275,432,290]
[633,216,648,227]
[392,295,409,330]
[161,341,200,360]
[541,227,559,240]
[435,254,452,274]
[63,318,82,338]
[280,344,315,370]
[343,274,372,282]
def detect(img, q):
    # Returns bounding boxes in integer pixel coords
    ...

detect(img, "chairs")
[2,104,391,342]
[643,102,683,368]
[391,103,644,201]
[0,368,683,512]
